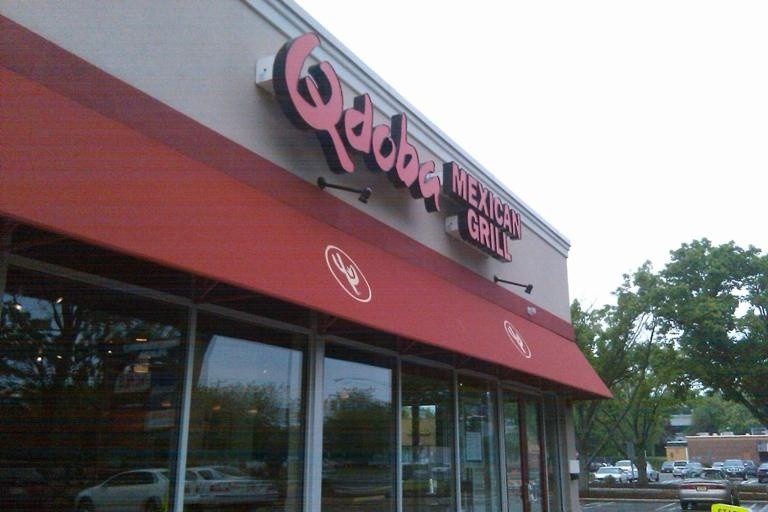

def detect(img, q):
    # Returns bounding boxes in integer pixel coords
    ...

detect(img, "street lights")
[331,374,390,393]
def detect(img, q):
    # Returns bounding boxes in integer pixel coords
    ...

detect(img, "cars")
[75,466,201,512]
[679,469,739,509]
[181,463,542,512]
[593,459,767,485]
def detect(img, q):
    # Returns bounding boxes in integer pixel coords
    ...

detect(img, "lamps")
[318,177,371,203]
[494,274,533,294]
